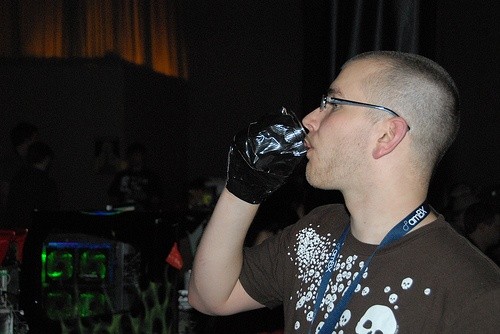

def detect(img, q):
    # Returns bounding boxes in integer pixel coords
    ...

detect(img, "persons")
[0,120,169,230]
[187,51,500,333]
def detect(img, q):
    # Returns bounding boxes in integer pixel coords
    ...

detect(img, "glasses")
[319,94,411,132]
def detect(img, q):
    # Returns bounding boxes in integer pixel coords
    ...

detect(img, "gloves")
[225,109,310,204]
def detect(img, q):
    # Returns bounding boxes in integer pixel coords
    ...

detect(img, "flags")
[166,242,185,269]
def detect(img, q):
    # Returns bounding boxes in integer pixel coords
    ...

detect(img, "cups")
[249,110,311,165]
